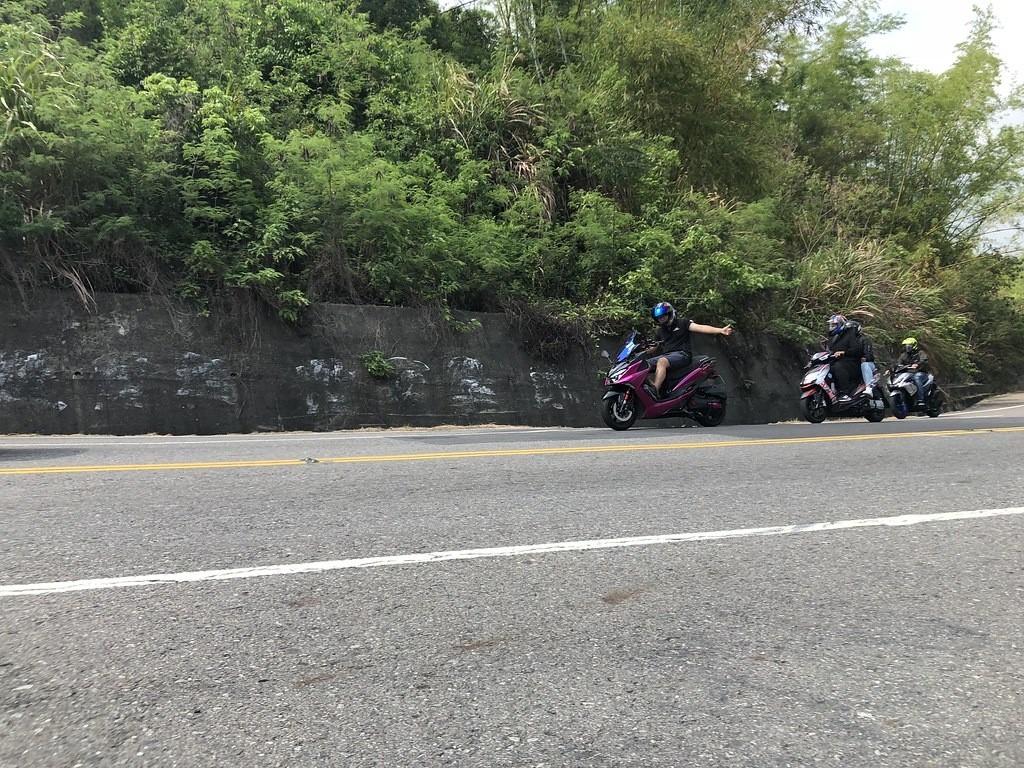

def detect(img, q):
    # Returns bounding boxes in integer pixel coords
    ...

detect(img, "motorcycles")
[880,362,944,420]
[800,348,892,424]
[601,329,728,432]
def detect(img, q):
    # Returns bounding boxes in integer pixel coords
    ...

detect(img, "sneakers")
[640,384,660,399]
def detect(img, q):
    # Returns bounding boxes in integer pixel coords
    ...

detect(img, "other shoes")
[838,394,852,401]
[862,387,873,398]
[917,401,925,405]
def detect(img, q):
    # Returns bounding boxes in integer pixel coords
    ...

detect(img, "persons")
[641,301,733,402]
[825,314,876,401]
[896,338,931,406]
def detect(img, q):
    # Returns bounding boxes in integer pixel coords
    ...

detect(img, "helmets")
[826,314,845,337]
[652,302,675,328]
[902,337,918,353]
[844,320,861,338]
